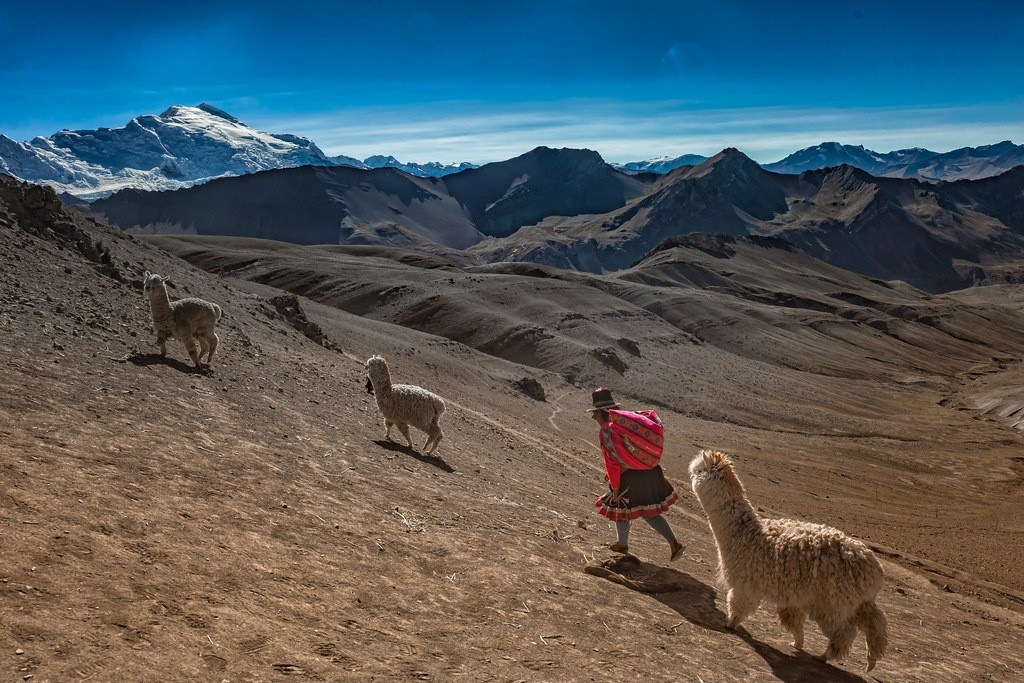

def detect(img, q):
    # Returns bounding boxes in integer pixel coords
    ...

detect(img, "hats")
[587,387,621,412]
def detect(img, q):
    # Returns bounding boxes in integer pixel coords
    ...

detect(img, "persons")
[585,386,686,561]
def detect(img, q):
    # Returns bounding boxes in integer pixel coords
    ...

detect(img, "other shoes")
[607,542,628,553]
[670,543,684,562]
